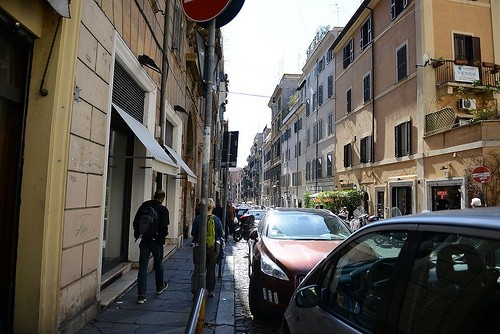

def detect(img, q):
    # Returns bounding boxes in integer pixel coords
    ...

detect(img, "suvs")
[248,208,379,319]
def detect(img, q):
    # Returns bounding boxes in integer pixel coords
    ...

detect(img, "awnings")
[165,144,198,183]
[112,103,180,175]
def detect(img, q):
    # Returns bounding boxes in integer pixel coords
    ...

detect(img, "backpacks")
[198,215,215,248]
[138,201,164,240]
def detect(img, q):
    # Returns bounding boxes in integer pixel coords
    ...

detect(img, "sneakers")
[137,296,146,303]
[156,282,168,294]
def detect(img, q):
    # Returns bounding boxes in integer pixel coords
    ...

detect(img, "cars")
[233,203,264,224]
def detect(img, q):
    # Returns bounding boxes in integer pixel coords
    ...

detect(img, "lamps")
[174,105,188,113]
[138,55,161,73]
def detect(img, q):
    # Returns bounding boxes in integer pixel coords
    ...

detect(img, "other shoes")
[209,292,215,296]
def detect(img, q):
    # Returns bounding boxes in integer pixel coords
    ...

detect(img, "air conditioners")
[460,99,477,110]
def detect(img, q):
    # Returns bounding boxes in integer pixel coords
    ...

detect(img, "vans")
[283,207,500,334]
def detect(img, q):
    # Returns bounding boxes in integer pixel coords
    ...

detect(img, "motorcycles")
[233,213,255,242]
[353,208,378,226]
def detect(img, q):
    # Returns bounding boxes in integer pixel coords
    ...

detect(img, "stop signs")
[472,167,492,185]
[181,0,230,23]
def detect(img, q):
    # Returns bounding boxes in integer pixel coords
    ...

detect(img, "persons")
[226,201,235,235]
[133,190,170,304]
[211,203,224,223]
[191,198,223,298]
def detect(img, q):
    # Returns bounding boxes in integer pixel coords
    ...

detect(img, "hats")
[470,198,482,207]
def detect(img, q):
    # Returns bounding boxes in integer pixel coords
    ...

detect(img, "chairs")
[363,244,500,334]
